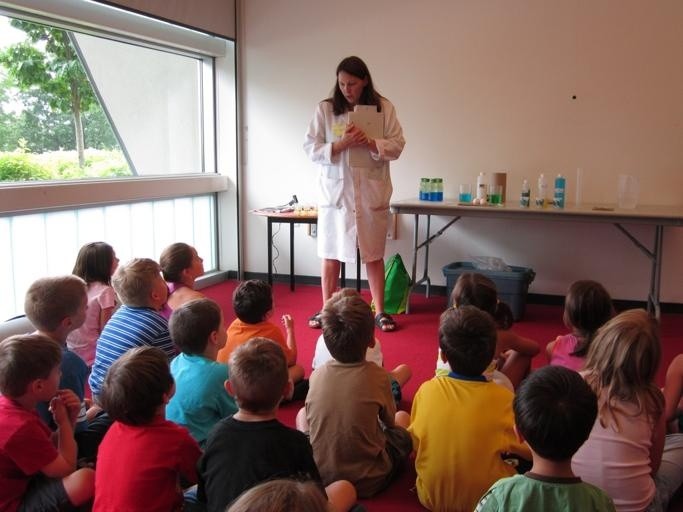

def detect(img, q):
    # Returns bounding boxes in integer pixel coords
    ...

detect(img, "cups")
[459,183,472,204]
[617,172,639,208]
[487,184,503,206]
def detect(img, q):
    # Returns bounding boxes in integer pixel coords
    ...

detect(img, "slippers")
[375,313,397,332]
[307,312,324,329]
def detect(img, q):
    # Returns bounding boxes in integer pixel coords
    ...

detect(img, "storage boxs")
[443,262,535,323]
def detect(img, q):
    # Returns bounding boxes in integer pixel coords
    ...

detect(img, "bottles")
[430,178,443,201]
[536,174,547,209]
[476,173,486,200]
[519,181,529,209]
[418,178,430,201]
[552,174,566,209]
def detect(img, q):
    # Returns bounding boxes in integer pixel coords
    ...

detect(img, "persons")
[474,365,616,512]
[195,338,357,512]
[409,304,535,512]
[533,280,616,371]
[0,331,96,511]
[161,299,238,452]
[346,103,384,168]
[304,55,404,334]
[567,309,670,511]
[306,287,412,498]
[91,345,201,512]
[66,240,120,366]
[449,272,512,317]
[25,276,89,424]
[91,257,176,418]
[158,242,206,304]
[219,280,310,410]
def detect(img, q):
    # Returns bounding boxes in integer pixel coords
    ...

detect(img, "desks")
[393,199,683,322]
[249,208,364,291]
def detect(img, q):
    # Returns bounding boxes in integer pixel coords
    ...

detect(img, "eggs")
[479,199,487,206]
[472,198,480,206]
